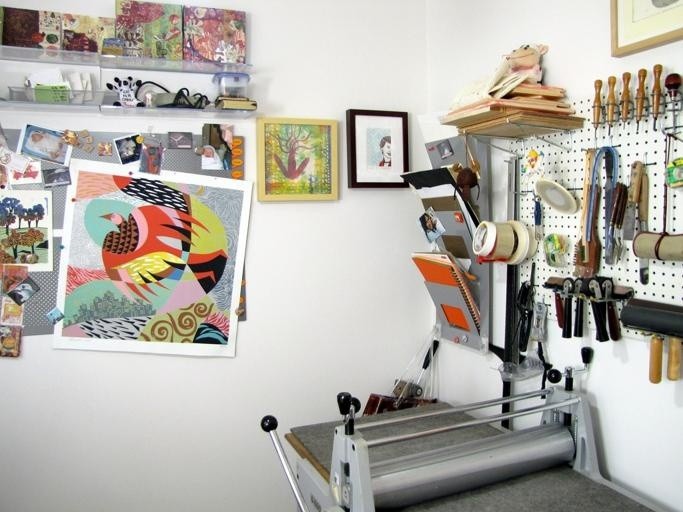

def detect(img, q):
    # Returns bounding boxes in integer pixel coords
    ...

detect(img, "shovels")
[622,160,643,240]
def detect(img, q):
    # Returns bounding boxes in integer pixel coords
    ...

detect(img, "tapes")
[474,220,537,264]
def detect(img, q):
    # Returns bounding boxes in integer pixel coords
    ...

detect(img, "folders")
[411,252,481,334]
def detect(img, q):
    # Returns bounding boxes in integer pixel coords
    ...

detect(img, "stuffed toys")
[505,43,547,84]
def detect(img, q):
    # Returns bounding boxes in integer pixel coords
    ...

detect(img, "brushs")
[572,147,600,277]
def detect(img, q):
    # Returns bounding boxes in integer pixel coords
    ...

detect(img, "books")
[434,65,586,140]
[399,158,481,341]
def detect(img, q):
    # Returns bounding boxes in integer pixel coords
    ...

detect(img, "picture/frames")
[610,0,683,57]
[255,116,339,202]
[346,109,412,187]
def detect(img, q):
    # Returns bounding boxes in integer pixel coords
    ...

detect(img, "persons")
[375,135,391,168]
